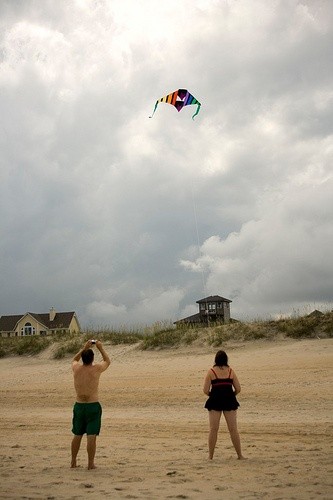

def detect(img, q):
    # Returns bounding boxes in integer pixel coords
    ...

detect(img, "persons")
[202,349,248,461]
[70,339,111,469]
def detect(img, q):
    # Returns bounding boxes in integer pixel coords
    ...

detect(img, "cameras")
[91,339,95,344]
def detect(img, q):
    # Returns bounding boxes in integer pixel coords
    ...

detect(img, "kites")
[149,87,202,123]
